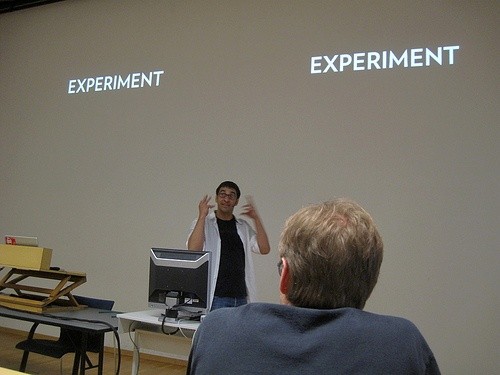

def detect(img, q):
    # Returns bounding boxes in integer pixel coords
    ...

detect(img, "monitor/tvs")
[148,247,212,314]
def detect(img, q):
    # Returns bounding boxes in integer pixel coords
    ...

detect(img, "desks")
[117,300,194,375]
[1,287,126,374]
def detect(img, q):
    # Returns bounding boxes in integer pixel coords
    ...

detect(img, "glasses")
[277,260,283,276]
[218,193,235,200]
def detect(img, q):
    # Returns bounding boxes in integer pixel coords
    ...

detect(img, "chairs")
[14,294,118,374]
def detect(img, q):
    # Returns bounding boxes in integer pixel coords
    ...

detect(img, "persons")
[186,197,443,374]
[187,181,270,310]
